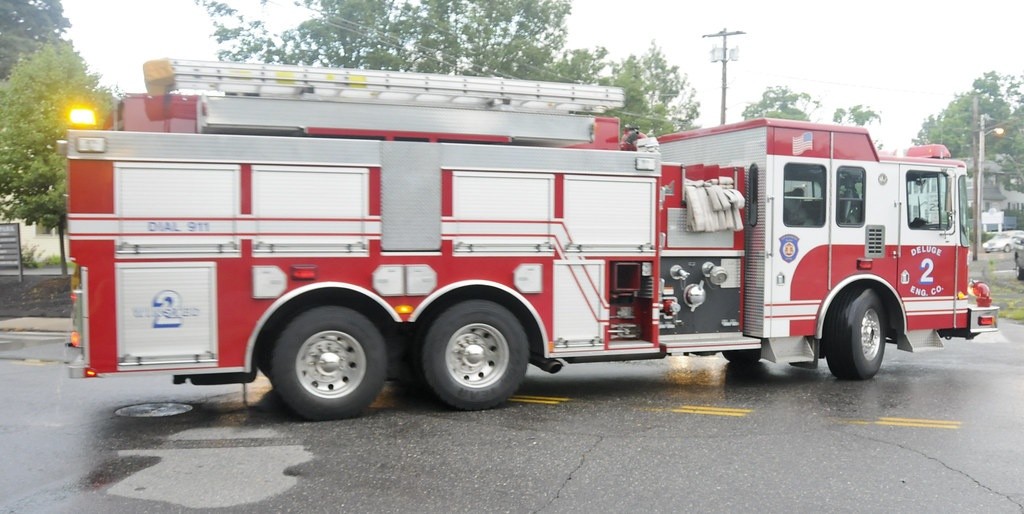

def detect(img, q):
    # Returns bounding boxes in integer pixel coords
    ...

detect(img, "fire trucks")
[60,56,1000,424]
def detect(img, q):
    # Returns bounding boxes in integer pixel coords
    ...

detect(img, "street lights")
[972,126,1004,261]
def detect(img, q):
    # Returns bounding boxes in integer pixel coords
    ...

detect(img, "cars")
[981,231,1024,253]
[1013,235,1024,280]
